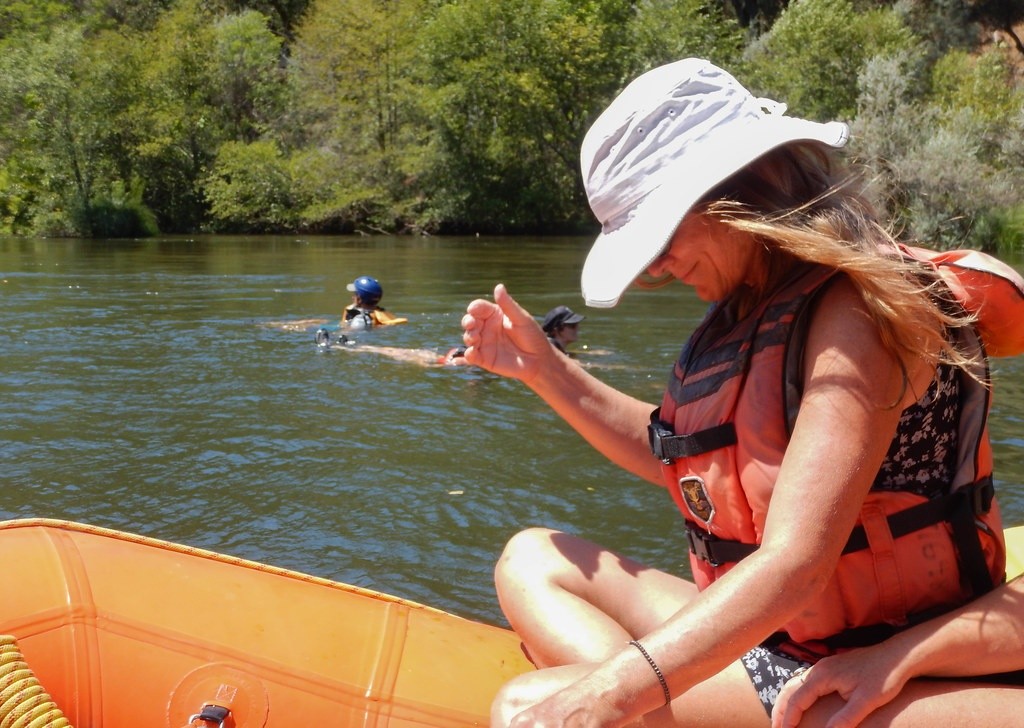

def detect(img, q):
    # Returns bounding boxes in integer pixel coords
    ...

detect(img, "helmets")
[346,276,382,305]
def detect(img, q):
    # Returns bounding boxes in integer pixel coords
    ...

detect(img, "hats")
[543,305,584,333]
[580,58,849,308]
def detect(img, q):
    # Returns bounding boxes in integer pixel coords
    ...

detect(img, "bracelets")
[629,639,671,705]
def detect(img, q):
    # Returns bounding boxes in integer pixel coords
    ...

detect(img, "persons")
[341,275,384,332]
[771,573,1024,726]
[542,304,587,354]
[463,56,1005,727]
